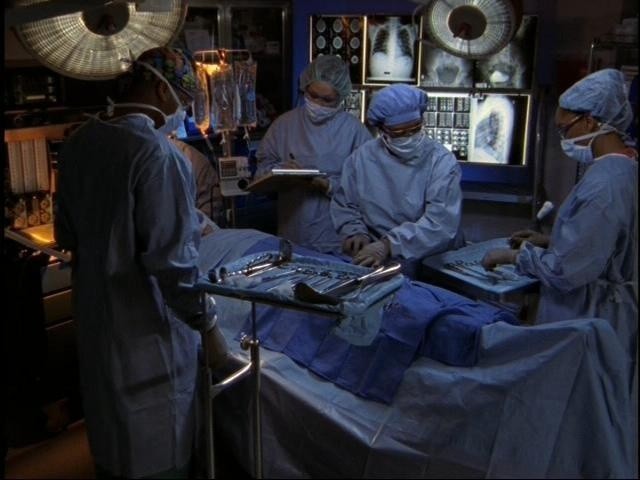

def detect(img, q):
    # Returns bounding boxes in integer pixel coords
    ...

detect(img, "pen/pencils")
[289,152,296,160]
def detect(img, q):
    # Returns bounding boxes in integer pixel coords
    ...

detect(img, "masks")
[380,125,427,159]
[156,109,186,136]
[304,98,341,123]
[561,140,593,164]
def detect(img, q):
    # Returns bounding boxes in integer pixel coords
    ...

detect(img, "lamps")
[15,0,189,82]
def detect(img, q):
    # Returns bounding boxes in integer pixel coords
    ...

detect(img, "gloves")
[510,230,550,248]
[353,239,389,268]
[344,235,369,256]
[482,248,516,271]
[201,324,227,371]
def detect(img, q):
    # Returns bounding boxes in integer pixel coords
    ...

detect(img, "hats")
[559,68,634,132]
[367,83,428,126]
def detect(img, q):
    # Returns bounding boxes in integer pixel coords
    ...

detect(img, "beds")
[196,326,487,480]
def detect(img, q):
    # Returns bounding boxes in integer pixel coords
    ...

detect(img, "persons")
[191,208,611,442]
[330,82,463,281]
[52,47,230,478]
[249,55,377,259]
[167,134,227,229]
[480,67,639,379]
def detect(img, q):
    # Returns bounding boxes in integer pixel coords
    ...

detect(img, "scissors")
[448,260,498,281]
[246,263,363,293]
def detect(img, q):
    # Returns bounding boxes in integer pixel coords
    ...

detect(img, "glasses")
[381,120,426,138]
[554,114,584,139]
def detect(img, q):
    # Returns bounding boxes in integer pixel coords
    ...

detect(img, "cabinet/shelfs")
[3,246,77,342]
[422,237,542,326]
[575,39,640,184]
